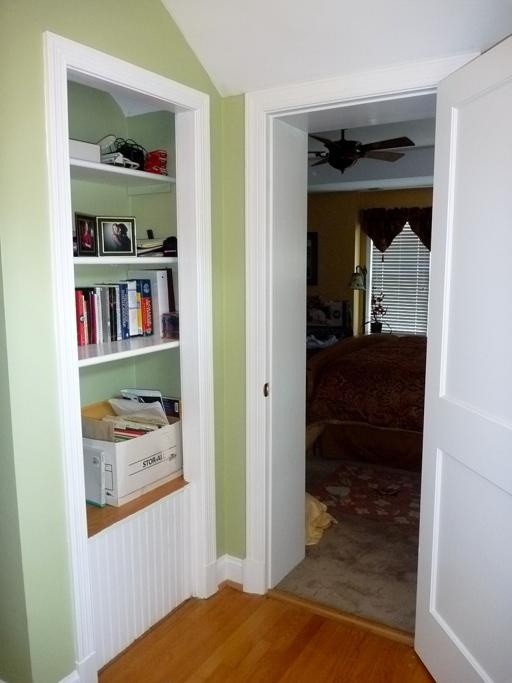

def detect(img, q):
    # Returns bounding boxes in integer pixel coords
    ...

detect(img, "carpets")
[305,458,420,530]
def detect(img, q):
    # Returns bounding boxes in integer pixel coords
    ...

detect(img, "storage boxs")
[83,415,184,508]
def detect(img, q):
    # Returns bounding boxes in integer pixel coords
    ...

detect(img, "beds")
[307,331,427,473]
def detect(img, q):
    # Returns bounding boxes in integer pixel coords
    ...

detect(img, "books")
[75,267,175,346]
[100,389,179,442]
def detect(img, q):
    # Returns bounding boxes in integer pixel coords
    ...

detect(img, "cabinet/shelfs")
[42,30,217,676]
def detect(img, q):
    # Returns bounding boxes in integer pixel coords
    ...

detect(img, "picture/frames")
[74,212,137,256]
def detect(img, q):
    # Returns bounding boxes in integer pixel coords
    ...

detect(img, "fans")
[308,129,416,174]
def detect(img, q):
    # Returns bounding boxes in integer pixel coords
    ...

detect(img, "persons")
[112,223,131,251]
[80,220,93,251]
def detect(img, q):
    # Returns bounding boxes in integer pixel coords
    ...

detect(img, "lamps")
[347,264,368,334]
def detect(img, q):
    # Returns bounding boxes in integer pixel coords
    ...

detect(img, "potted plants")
[361,291,392,334]
